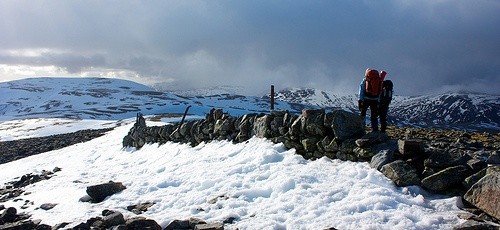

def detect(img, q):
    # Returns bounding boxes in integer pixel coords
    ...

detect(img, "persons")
[358,68,382,133]
[379,79,393,133]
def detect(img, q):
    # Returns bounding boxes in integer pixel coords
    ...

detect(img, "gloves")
[358,100,362,110]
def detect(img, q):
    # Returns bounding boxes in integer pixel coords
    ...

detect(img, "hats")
[365,66,370,72]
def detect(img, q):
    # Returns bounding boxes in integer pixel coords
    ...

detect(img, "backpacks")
[382,80,393,104]
[365,70,380,98]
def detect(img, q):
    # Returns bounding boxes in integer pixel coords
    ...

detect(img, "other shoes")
[380,130,386,134]
[372,130,378,133]
